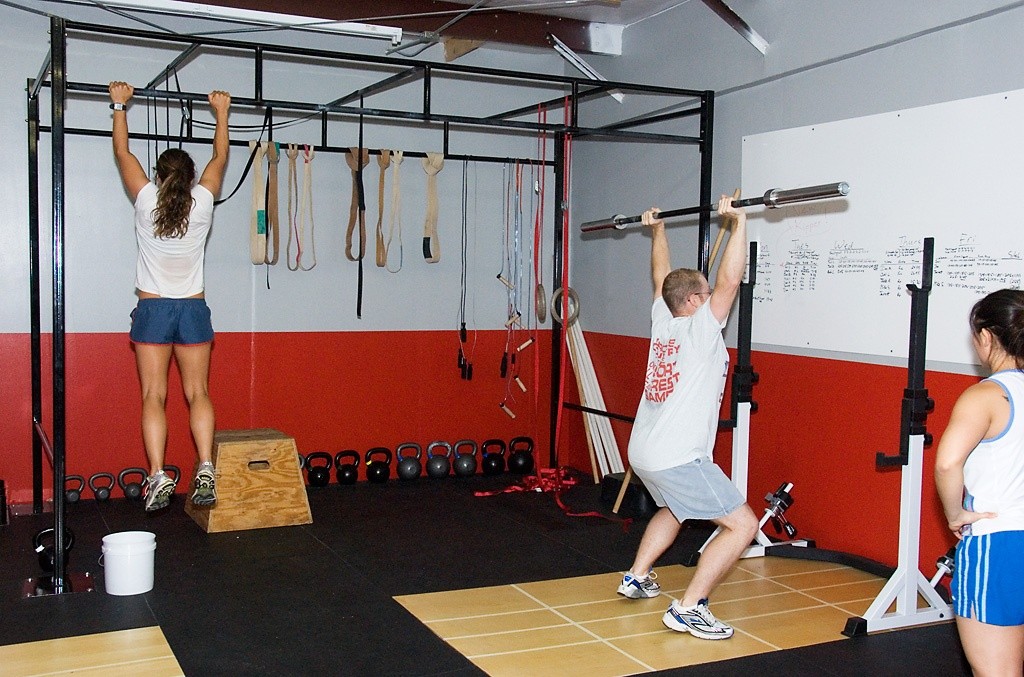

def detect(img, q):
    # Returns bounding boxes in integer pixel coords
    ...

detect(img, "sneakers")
[617,567,662,598]
[662,597,734,640]
[143,470,175,511]
[191,462,217,506]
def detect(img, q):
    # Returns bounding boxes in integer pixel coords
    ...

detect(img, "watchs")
[109,104,127,112]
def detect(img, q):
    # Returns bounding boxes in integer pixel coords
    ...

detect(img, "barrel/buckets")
[98,531,157,596]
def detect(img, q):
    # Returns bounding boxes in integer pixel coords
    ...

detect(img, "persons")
[615,192,758,640]
[935,289,1024,677]
[107,80,229,512]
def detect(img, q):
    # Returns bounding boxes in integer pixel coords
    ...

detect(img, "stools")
[184,426,314,533]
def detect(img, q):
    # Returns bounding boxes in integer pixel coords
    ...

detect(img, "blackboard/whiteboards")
[723,88,1024,378]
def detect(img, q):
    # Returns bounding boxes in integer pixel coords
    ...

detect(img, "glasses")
[688,288,713,301]
